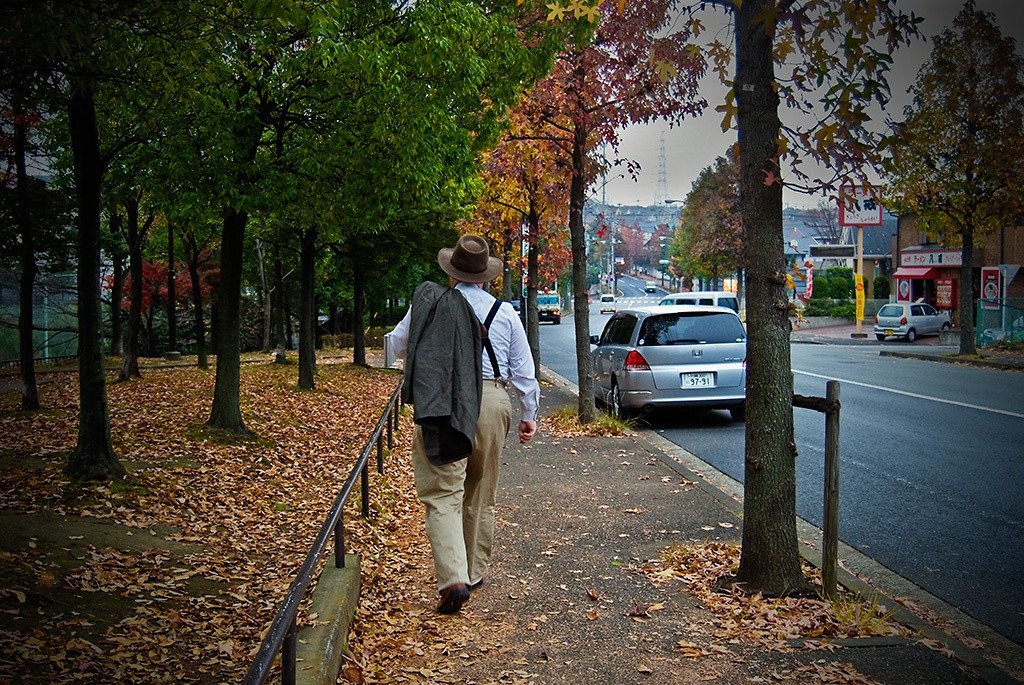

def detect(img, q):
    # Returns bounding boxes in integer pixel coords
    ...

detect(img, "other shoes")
[437,582,469,614]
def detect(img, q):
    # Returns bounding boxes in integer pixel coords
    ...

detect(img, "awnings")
[892,268,940,279]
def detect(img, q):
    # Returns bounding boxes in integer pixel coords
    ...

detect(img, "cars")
[590,305,746,422]
[980,316,1024,349]
[873,302,952,343]
[511,300,521,316]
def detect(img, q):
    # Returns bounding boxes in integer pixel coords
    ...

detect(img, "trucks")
[538,288,564,324]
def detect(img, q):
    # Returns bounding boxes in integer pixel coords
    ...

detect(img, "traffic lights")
[522,275,528,298]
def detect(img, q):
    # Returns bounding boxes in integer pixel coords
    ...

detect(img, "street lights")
[664,199,707,291]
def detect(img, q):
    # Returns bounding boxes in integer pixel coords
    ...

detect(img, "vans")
[656,291,741,320]
[599,294,618,314]
[645,281,658,293]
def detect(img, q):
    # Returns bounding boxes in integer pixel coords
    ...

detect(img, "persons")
[389,234,542,615]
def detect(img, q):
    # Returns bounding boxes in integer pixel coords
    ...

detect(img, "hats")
[437,234,504,283]
